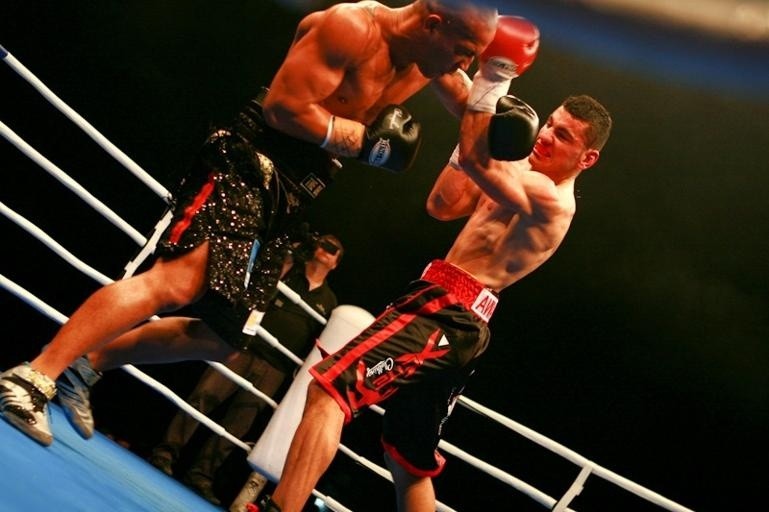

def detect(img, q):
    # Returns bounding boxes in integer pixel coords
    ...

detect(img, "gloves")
[320,104,423,176]
[466,14,541,162]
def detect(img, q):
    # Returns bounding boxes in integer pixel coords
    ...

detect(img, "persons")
[2,1,540,447]
[246,16,610,512]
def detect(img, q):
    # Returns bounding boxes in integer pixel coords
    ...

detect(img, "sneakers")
[150,456,173,475]
[187,473,221,506]
[0,354,103,444]
[246,494,281,512]
